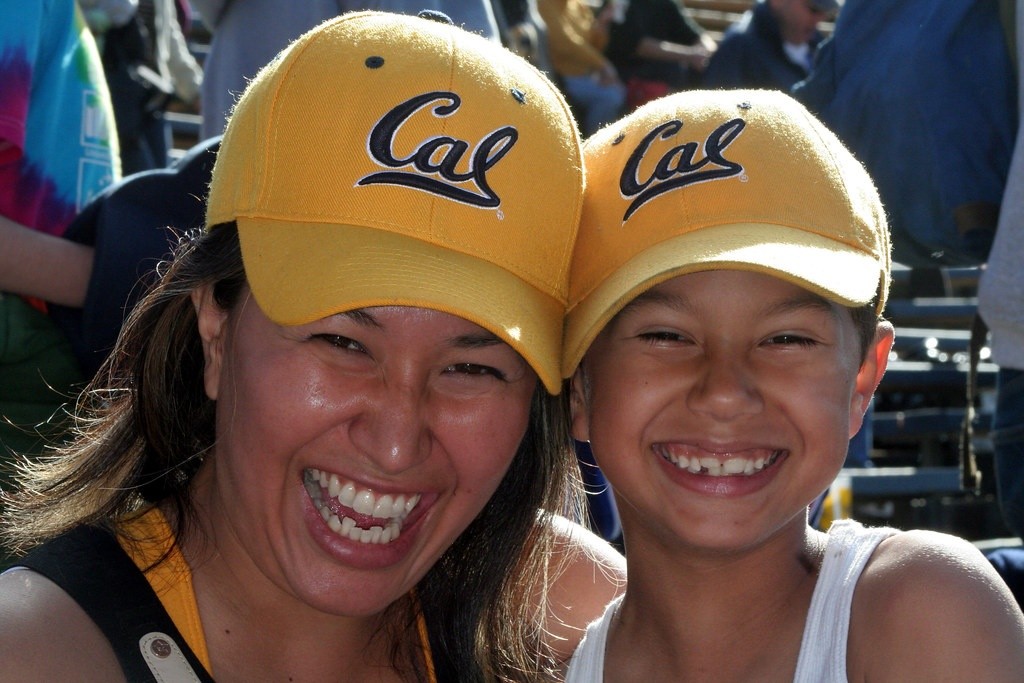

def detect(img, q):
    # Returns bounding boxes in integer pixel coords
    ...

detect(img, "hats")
[204,10,569,396]
[563,89,895,372]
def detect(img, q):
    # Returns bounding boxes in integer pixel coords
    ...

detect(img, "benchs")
[838,265,1024,553]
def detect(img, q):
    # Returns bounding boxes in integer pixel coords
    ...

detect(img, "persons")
[0,12,628,683]
[0,0,1024,571]
[562,90,1024,683]
